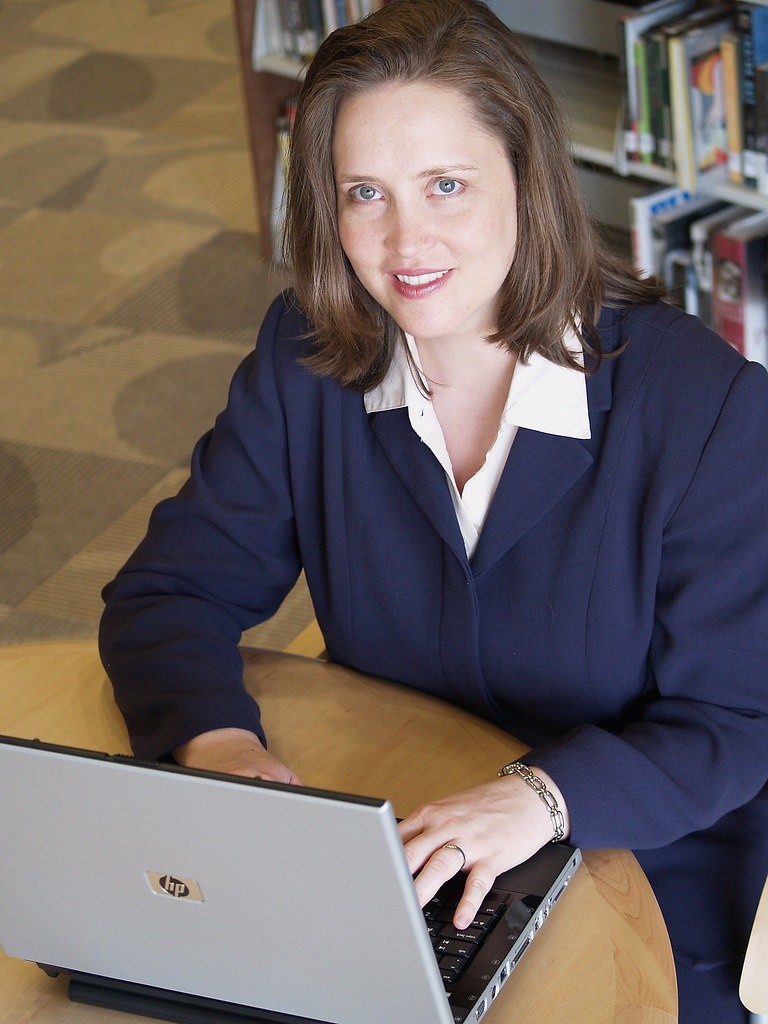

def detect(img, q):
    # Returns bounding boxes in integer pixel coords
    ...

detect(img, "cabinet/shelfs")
[234,1,768,268]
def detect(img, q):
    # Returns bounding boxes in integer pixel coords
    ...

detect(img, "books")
[617,1,768,370]
[251,0,383,263]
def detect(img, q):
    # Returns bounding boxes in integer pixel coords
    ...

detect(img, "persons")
[98,0,768,1024]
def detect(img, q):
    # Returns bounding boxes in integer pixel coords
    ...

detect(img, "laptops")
[0,735,582,1024]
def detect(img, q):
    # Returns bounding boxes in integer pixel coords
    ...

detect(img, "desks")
[1,640,679,1024]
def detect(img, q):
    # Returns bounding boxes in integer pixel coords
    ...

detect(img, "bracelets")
[497,762,564,843]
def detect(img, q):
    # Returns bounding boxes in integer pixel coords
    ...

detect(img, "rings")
[443,845,466,870]
